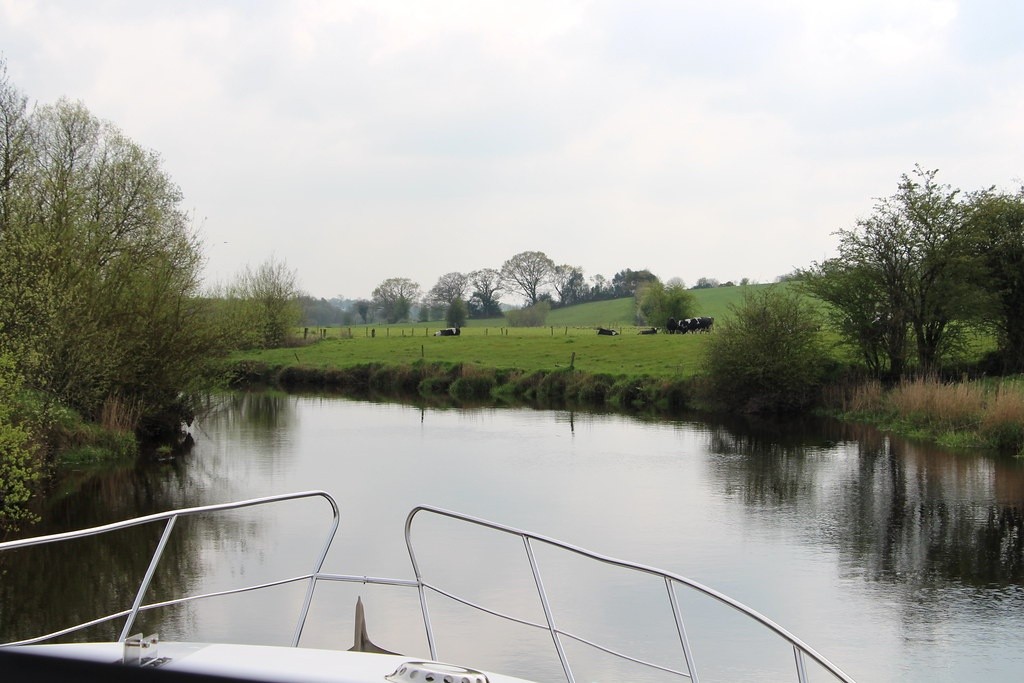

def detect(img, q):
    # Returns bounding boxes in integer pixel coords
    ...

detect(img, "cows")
[598,329,619,335]
[434,328,460,336]
[637,327,661,335]
[666,317,714,334]
